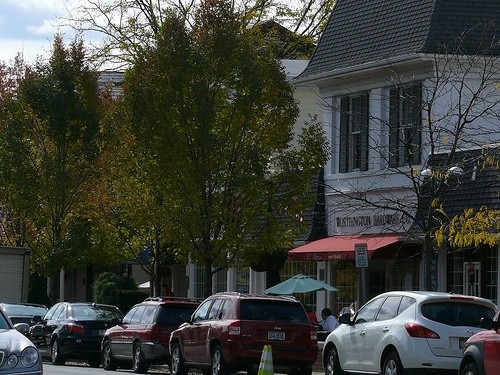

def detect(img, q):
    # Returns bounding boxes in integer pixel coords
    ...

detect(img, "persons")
[303,305,317,322]
[321,308,339,332]
[161,284,174,301]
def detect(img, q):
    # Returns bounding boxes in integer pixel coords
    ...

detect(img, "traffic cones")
[257,345,274,375]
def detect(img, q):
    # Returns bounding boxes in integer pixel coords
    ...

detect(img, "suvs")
[100,296,205,374]
[169,292,319,375]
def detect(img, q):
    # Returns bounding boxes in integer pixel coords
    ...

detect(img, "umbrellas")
[264,273,339,294]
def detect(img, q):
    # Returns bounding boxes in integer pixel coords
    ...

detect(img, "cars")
[0,306,43,375]
[0,302,49,327]
[458,307,500,375]
[322,289,498,375]
[28,301,125,366]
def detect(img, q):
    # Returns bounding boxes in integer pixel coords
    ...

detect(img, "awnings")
[120,248,153,264]
[287,235,403,262]
